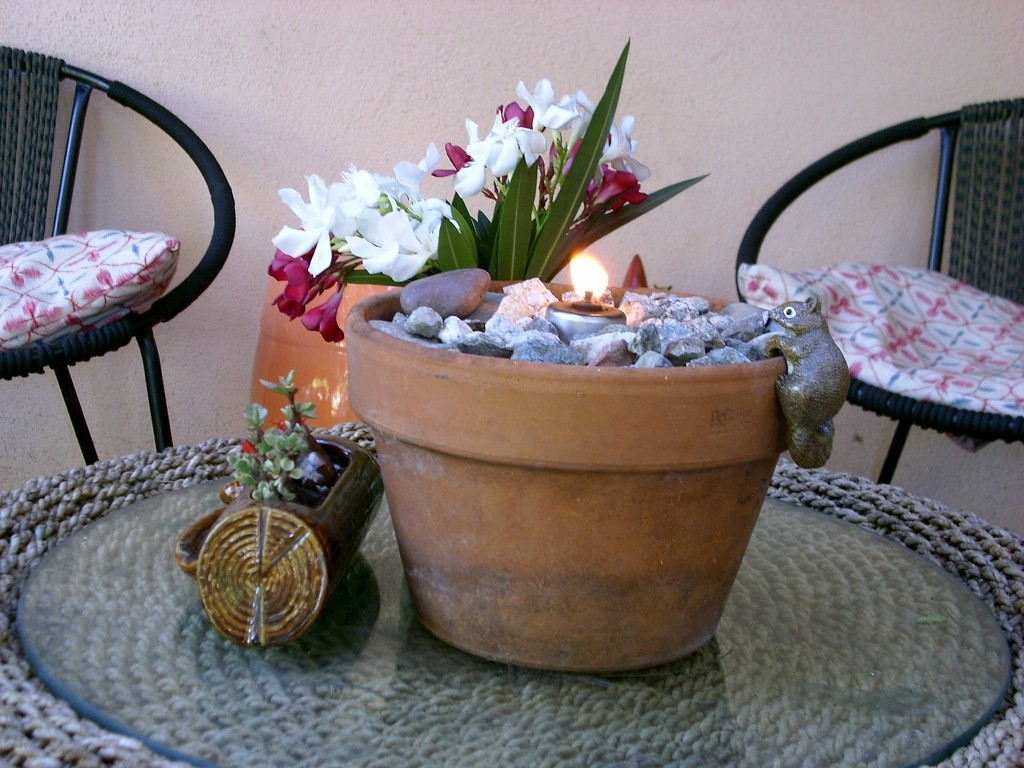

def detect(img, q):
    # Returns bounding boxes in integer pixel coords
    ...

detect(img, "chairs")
[735,98,1023,485]
[0,46,236,466]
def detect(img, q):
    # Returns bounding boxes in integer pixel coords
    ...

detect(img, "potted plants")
[173,369,386,647]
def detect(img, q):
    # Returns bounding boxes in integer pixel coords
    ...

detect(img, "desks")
[0,421,1024,768]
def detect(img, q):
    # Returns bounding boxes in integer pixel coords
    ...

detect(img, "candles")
[546,249,628,341]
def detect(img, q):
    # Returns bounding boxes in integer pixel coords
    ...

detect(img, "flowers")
[267,37,713,344]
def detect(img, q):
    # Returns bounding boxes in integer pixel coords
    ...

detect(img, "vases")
[345,280,789,673]
